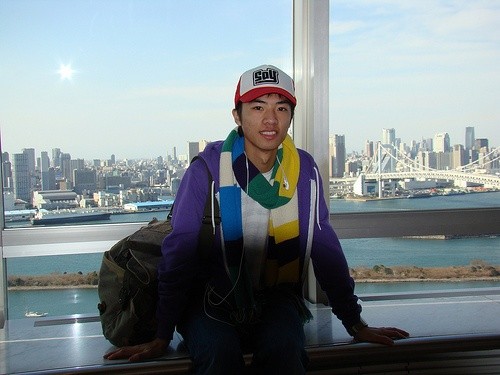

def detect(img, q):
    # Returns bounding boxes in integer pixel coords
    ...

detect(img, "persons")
[102,65,410,375]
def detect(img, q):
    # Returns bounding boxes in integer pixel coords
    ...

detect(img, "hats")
[235,64,297,109]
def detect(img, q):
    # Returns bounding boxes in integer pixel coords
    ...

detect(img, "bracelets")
[345,318,369,336]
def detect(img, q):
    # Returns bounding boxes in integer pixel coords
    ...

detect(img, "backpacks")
[98,216,174,348]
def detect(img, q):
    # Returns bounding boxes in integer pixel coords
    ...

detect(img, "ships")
[31,209,112,225]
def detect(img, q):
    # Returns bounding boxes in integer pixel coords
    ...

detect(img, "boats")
[25,311,48,318]
[406,193,429,199]
